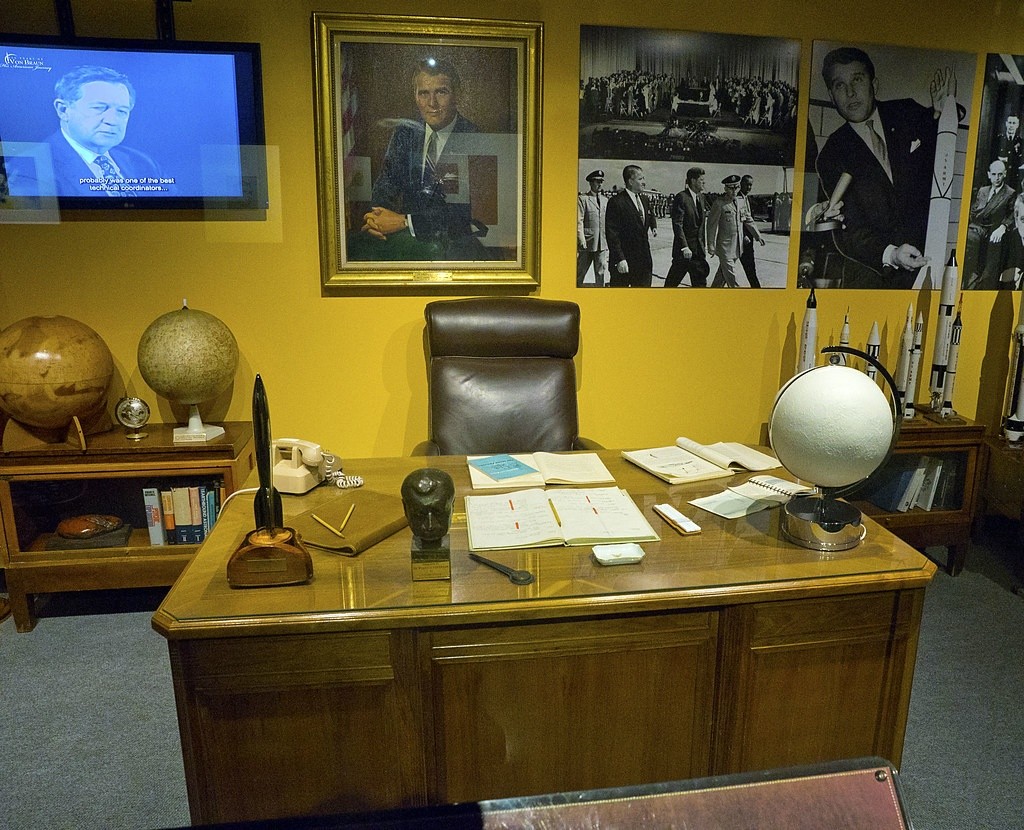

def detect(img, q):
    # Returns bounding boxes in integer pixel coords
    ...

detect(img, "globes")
[768,344,901,552]
[0,315,115,453]
[137,298,240,444]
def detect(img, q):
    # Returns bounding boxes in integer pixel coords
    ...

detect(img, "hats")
[585,169,606,181]
[721,174,741,188]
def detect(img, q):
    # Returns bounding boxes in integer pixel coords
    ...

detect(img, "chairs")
[412,297,608,457]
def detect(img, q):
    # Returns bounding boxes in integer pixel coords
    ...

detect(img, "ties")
[94,156,136,197]
[634,193,644,225]
[419,132,441,192]
[694,194,704,222]
[1008,136,1015,151]
[744,196,751,212]
[732,198,739,209]
[595,193,600,205]
[988,188,997,203]
[864,120,894,184]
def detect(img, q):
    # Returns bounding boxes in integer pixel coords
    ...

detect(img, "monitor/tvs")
[0,31,269,211]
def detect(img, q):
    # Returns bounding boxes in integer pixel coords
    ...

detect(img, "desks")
[151,446,938,830]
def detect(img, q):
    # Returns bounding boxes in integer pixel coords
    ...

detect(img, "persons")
[816,45,957,287]
[578,164,791,289]
[401,468,456,541]
[3,67,177,197]
[578,68,798,127]
[962,112,1024,290]
[346,59,484,261]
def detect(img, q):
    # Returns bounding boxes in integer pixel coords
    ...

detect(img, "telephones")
[269,438,344,495]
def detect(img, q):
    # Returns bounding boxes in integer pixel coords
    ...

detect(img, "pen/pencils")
[340,504,355,533]
[311,514,345,539]
[549,499,561,527]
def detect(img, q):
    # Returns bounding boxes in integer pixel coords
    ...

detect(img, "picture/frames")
[310,8,546,292]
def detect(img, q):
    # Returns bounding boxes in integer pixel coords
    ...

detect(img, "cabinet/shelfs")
[847,404,1024,575]
[1,421,256,634]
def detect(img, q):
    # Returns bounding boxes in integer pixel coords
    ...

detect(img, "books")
[466,450,616,489]
[463,486,661,552]
[142,475,227,546]
[620,437,782,485]
[689,474,814,519]
